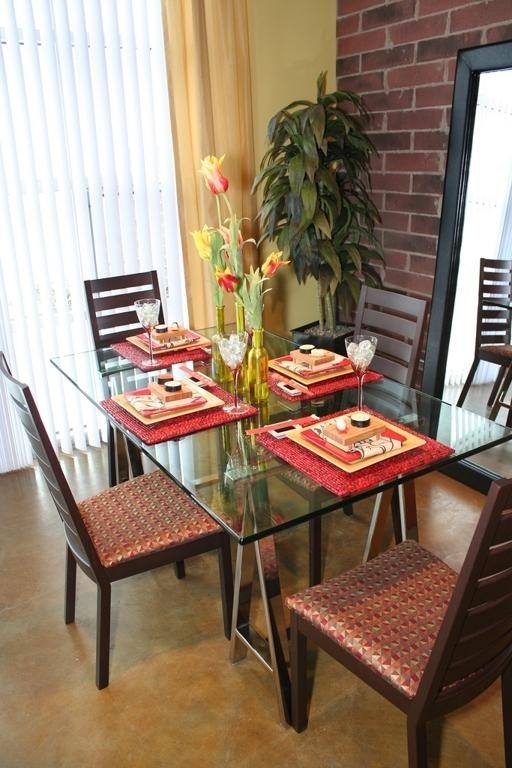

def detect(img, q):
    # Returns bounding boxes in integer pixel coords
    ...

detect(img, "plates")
[112,378,226,425]
[266,349,354,385]
[287,410,427,473]
[125,328,212,354]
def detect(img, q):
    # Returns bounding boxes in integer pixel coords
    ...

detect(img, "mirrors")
[420,39,511,495]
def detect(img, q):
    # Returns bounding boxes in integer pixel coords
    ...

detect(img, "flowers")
[190,153,291,330]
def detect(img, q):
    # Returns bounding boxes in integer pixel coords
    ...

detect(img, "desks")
[50,322,511,728]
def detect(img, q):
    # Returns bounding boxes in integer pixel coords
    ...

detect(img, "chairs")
[455,258,511,426]
[283,479,510,762]
[85,271,168,486]
[0,349,234,690]
[269,286,430,587]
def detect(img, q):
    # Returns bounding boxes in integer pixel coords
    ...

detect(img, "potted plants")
[250,71,386,416]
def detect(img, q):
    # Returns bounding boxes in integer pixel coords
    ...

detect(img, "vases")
[213,306,270,408]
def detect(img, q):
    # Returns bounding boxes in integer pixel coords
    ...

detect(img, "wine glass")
[216,331,250,413]
[134,298,163,366]
[345,336,376,412]
